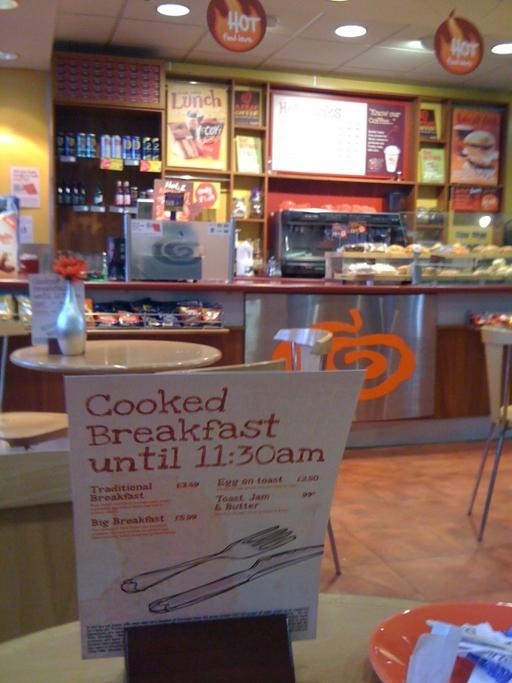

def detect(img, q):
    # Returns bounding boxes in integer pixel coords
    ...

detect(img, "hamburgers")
[463,130,500,179]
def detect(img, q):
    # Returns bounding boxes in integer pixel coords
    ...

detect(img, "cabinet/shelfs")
[448,97,510,246]
[51,51,163,271]
[164,63,231,225]
[415,95,449,244]
[231,77,268,278]
[265,81,417,278]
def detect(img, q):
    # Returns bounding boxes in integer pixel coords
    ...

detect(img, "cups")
[384,144,400,173]
[416,205,447,224]
[232,196,246,220]
[455,124,471,158]
[132,186,154,206]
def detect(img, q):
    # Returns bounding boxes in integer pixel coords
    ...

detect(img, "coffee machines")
[272,205,409,274]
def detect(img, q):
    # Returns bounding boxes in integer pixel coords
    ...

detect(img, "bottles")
[248,185,266,218]
[54,175,131,208]
[266,256,280,283]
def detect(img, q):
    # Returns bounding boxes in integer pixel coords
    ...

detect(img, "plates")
[369,601,512,682]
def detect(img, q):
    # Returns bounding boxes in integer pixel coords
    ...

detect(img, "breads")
[344,240,512,280]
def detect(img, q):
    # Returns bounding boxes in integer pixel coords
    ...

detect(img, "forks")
[148,544,326,615]
[113,522,298,594]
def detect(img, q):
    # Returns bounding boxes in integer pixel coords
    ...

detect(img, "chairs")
[0,318,69,450]
[467,344,511,541]
[156,360,341,579]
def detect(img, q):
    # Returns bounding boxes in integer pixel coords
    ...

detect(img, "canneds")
[130,187,154,207]
[55,132,160,161]
